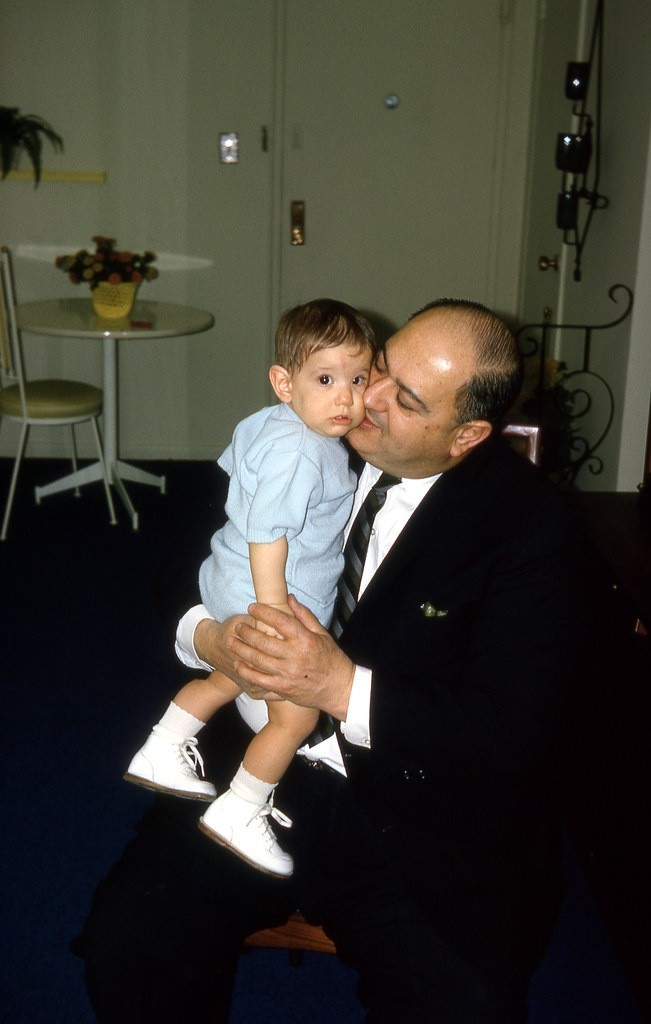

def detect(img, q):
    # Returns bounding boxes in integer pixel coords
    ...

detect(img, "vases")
[89,279,139,320]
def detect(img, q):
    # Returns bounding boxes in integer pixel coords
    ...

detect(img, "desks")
[8,299,214,529]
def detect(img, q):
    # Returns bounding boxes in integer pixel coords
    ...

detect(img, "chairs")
[0,245,118,541]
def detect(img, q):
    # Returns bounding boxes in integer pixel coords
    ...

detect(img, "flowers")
[55,236,160,290]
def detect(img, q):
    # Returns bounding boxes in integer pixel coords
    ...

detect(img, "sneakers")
[122,731,219,803]
[197,788,297,880]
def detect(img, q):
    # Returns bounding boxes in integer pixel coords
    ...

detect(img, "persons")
[124,301,375,878]
[78,298,568,1023]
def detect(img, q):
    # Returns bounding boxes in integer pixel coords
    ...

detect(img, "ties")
[306,469,402,749]
